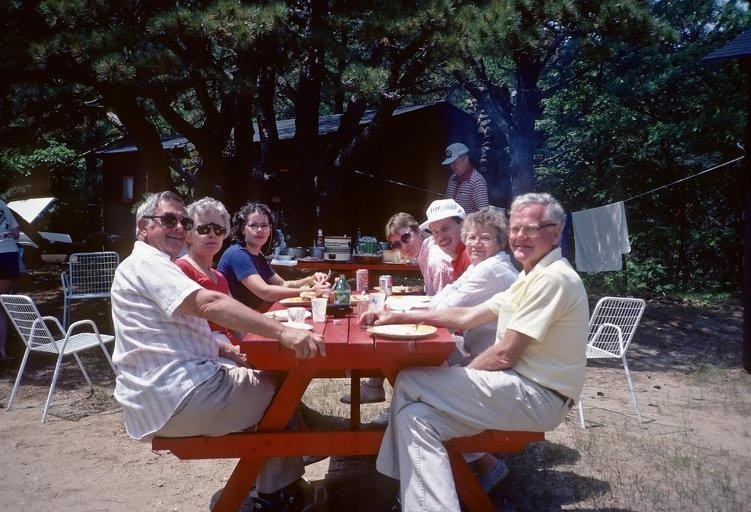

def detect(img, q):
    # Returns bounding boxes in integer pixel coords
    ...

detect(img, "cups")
[356,300,372,330]
[369,292,385,313]
[310,297,328,323]
[287,307,307,323]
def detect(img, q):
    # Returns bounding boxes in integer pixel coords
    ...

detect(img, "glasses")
[506,223,558,239]
[390,231,412,251]
[196,223,226,236]
[144,215,195,231]
[247,222,273,231]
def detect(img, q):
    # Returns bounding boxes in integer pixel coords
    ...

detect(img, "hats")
[418,199,466,231]
[441,143,469,165]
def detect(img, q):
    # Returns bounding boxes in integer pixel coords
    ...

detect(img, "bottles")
[332,274,351,315]
[315,228,323,247]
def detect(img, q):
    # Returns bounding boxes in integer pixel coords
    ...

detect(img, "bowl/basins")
[310,247,324,259]
[354,235,405,266]
[283,246,304,258]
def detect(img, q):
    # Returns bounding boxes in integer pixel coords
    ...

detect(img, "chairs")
[576,297,646,430]
[61,251,119,329]
[0,294,117,423]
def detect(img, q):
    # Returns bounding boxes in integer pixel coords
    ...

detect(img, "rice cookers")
[322,235,352,261]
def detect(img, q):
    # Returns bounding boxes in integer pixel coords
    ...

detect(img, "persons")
[2,202,21,295]
[429,205,522,495]
[109,191,341,511]
[426,197,471,274]
[443,143,488,212]
[343,213,456,403]
[359,190,588,512]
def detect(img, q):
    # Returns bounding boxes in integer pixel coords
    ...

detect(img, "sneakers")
[253,481,344,512]
[478,460,510,492]
[340,381,386,404]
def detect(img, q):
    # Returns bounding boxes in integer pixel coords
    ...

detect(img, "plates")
[276,255,294,260]
[279,319,314,331]
[373,286,420,293]
[278,296,328,306]
[262,308,311,321]
[345,294,368,306]
[365,323,437,339]
[294,258,324,263]
[301,291,329,299]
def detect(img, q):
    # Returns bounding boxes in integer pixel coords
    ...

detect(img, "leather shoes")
[302,474,398,512]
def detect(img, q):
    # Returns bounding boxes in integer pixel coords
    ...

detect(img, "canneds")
[355,268,369,292]
[378,275,392,297]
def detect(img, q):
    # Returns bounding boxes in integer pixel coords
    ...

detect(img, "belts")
[549,389,574,409]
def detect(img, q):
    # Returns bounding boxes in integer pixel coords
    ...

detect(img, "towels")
[571,200,632,274]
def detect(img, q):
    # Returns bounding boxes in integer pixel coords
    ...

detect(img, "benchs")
[455,430,542,453]
[153,433,255,461]
